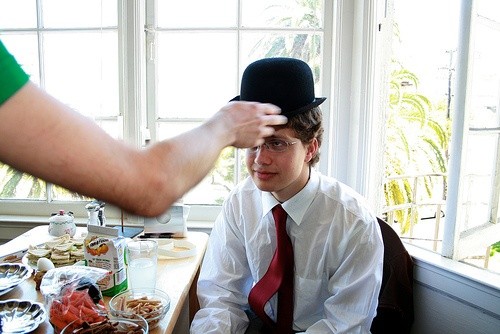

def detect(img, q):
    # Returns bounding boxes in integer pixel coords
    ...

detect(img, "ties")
[248,204,295,334]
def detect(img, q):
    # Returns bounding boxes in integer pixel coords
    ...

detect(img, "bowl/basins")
[108,287,171,331]
[60,310,150,334]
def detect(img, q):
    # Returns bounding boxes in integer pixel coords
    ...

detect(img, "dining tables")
[0,224,209,334]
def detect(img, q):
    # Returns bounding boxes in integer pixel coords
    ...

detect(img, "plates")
[22,246,44,275]
[0,299,46,334]
[0,263,34,297]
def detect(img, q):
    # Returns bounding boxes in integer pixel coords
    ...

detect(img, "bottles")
[85,202,101,227]
[98,202,106,227]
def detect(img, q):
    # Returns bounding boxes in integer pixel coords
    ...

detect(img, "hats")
[228,57,327,118]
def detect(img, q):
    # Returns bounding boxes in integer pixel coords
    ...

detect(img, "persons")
[188,56,385,333]
[1,40,289,218]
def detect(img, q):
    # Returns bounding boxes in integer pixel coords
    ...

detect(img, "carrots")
[50,290,107,333]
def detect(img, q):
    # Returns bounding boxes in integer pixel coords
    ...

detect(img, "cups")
[48,209,76,238]
[125,239,158,299]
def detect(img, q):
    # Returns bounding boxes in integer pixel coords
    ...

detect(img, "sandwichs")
[27,234,86,267]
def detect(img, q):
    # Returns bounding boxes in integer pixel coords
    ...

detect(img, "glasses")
[242,138,301,153]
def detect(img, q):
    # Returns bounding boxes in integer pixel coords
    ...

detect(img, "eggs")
[37,257,55,272]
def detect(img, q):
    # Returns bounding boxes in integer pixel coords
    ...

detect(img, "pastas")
[117,296,161,331]
